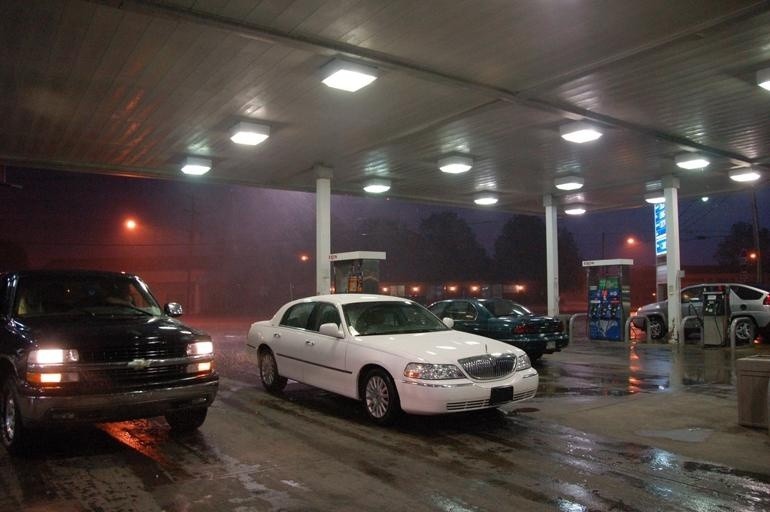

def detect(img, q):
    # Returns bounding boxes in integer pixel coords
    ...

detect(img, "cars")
[633,283,770,344]
[245,294,539,429]
[422,297,569,363]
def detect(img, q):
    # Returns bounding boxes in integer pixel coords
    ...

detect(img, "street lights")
[679,196,710,217]
[601,231,635,279]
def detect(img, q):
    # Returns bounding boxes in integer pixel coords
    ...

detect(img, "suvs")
[1,269,219,453]
